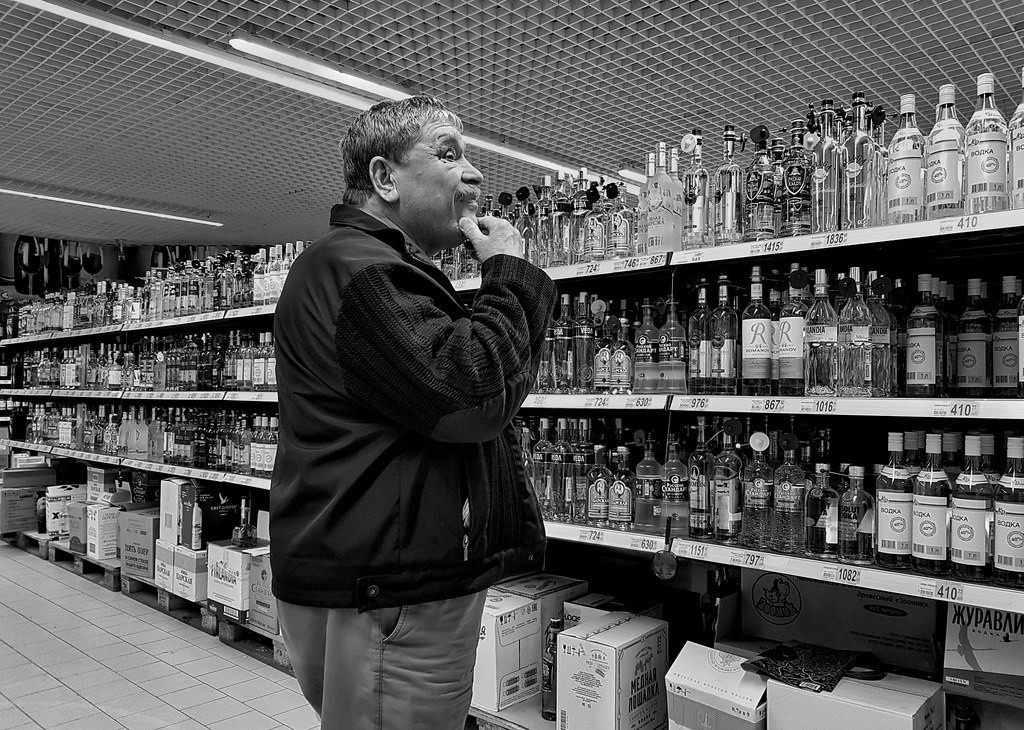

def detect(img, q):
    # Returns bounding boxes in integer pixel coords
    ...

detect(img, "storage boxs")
[470,569,946,730]
[0,459,280,638]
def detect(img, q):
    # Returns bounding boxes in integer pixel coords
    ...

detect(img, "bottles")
[513,417,1024,591]
[232,496,257,545]
[542,618,562,720]
[0,241,312,338]
[430,66,1024,280]
[530,266,1024,399]
[0,397,278,478]
[0,330,276,392]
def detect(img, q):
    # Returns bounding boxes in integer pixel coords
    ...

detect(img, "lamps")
[13,0,379,112]
[460,131,640,196]
[227,31,419,103]
[617,164,646,184]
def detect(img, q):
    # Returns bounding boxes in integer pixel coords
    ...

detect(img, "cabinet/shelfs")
[0,205,1024,730]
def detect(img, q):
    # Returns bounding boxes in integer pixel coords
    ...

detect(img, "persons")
[271,95,557,730]
